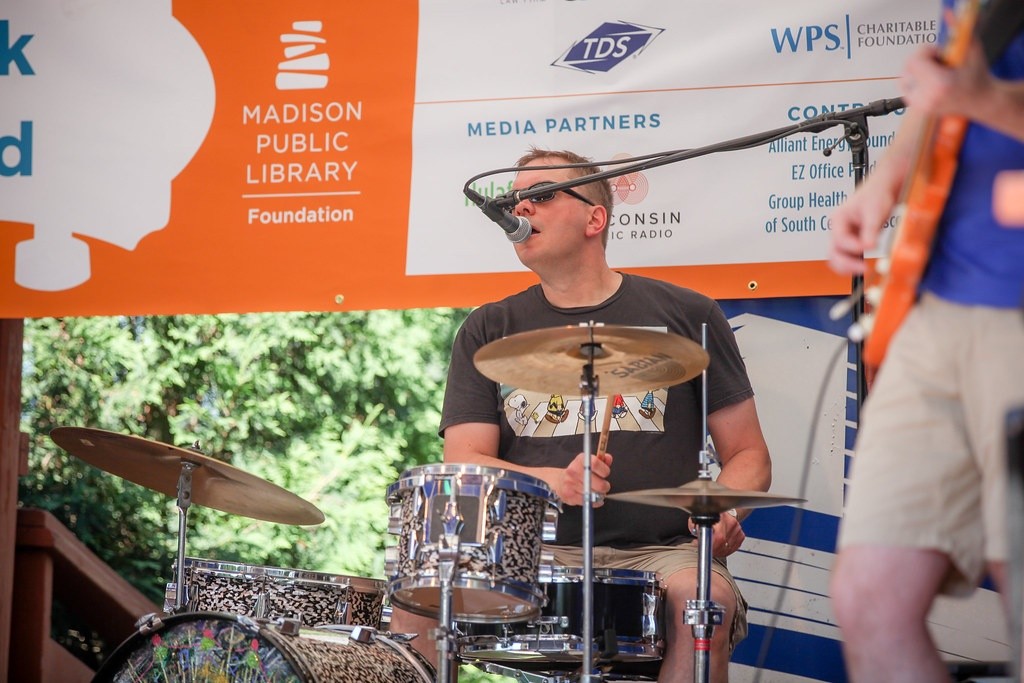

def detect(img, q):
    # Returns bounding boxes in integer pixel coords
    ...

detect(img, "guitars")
[847,0,978,371]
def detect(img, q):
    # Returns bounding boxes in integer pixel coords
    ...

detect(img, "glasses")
[526,182,595,214]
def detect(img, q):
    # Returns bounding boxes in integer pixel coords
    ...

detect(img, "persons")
[390,148,772,683]
[830,0,1024,683]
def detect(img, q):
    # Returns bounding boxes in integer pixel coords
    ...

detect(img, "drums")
[456,564,669,662]
[387,462,553,626]
[102,612,438,683]
[171,554,388,631]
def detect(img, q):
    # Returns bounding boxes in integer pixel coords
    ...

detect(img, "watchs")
[727,509,738,519]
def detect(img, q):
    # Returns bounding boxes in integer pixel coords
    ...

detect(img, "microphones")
[462,188,532,243]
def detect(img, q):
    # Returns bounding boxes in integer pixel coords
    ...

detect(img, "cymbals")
[472,325,711,399]
[603,478,809,510]
[47,425,327,527]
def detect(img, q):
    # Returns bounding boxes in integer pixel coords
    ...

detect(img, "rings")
[725,543,729,547]
